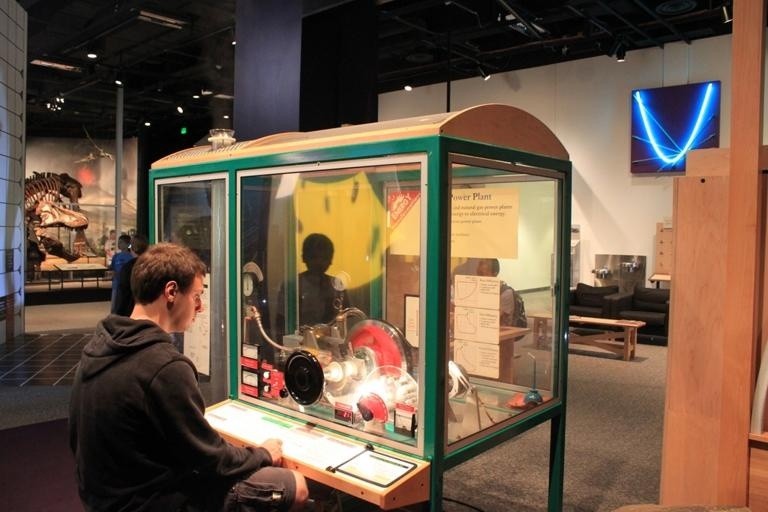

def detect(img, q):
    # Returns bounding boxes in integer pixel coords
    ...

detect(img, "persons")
[104,230,116,268]
[477,258,529,345]
[112,235,148,318]
[106,234,134,314]
[64,242,312,511]
[451,257,484,290]
[275,232,355,356]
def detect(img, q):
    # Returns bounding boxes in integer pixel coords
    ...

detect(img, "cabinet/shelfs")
[656,223,673,288]
[659,144,768,507]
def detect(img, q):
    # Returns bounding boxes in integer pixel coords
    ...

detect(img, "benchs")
[527,311,647,360]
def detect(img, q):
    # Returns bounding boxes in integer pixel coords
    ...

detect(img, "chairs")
[569,284,618,318]
[612,288,670,347]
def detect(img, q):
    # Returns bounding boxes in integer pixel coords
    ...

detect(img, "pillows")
[575,283,619,308]
[632,285,670,311]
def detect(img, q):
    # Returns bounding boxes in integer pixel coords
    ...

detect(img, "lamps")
[720,5,733,23]
[472,63,492,81]
[606,34,627,62]
